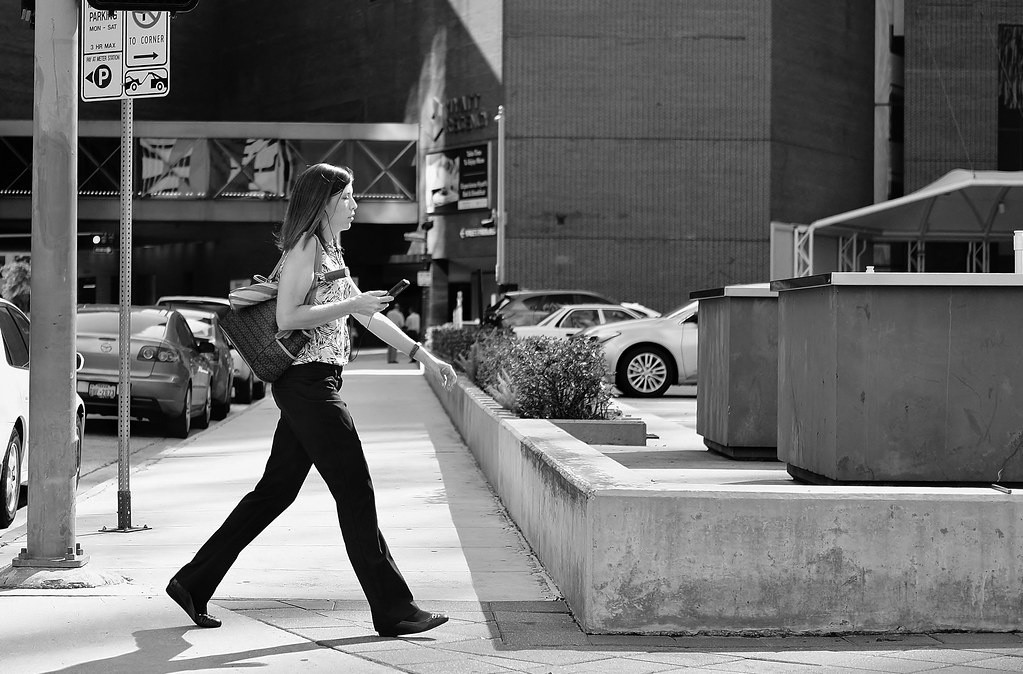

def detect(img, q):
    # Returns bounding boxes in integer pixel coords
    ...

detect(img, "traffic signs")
[81,0,172,103]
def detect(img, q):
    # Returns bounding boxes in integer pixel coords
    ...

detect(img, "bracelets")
[409,342,421,359]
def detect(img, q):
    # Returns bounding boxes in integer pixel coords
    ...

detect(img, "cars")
[155,293,268,421]
[511,302,647,349]
[0,297,87,528]
[76,303,217,440]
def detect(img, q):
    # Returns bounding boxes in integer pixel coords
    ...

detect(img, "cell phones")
[380,279,410,305]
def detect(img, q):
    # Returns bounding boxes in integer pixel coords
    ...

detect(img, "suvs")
[496,289,622,330]
[568,282,771,397]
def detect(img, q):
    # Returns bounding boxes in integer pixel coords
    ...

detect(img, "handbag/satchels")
[218,233,324,382]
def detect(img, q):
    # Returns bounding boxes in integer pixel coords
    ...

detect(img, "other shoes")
[379,612,449,639]
[166,577,222,626]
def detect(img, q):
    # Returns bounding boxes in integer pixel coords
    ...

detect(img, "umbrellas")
[228,267,350,311]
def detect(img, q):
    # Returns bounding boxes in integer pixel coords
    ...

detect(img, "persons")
[387,304,420,364]
[165,164,457,638]
